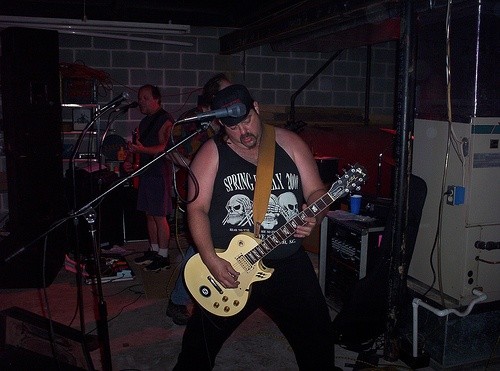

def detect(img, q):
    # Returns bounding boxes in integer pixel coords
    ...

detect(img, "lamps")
[0,9,195,48]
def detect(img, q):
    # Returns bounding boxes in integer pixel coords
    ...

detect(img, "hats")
[213,84,255,127]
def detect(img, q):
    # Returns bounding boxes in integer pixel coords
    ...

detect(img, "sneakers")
[134,251,160,265]
[145,254,172,271]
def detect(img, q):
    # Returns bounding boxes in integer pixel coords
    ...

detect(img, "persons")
[164,72,232,325]
[123,84,173,272]
[174,84,343,371]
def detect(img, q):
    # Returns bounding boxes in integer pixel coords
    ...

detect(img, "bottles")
[359,196,374,217]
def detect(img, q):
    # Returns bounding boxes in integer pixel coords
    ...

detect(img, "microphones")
[96,92,129,113]
[186,103,246,120]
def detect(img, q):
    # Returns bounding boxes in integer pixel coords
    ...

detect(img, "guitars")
[182,161,369,319]
[114,126,142,194]
[172,120,308,202]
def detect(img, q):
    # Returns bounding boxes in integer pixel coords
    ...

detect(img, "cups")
[351,195,362,213]
